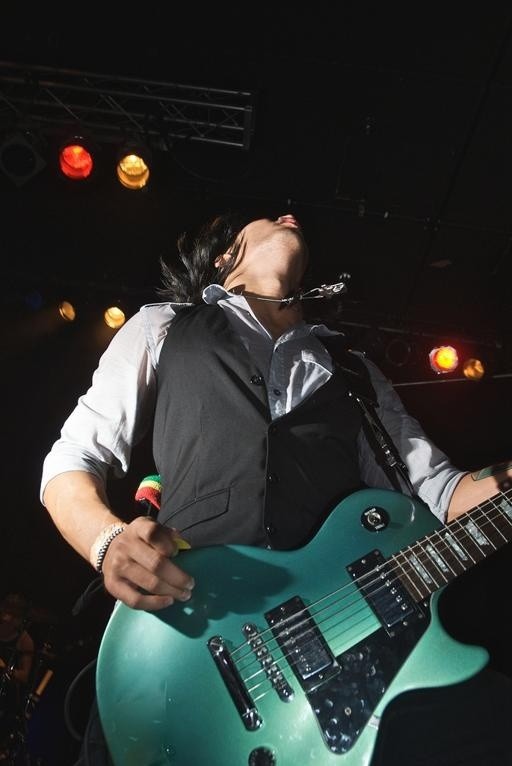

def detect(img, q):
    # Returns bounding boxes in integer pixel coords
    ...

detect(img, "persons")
[1,591,35,766]
[40,206,511,765]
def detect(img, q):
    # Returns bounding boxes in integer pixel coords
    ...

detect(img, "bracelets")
[88,518,129,576]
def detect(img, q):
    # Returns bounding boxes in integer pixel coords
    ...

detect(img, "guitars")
[95,481,510,764]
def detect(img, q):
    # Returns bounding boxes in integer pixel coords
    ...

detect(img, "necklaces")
[232,281,350,308]
